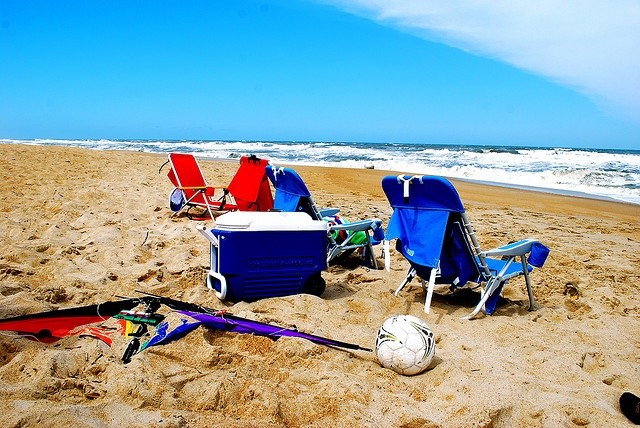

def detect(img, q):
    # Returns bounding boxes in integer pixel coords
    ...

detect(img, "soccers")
[373,315,435,377]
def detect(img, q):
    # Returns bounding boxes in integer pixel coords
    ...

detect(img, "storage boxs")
[196,210,330,303]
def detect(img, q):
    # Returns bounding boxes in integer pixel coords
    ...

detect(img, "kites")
[1,293,372,364]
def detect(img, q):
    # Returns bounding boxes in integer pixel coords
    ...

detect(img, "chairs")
[158,152,238,224]
[226,154,275,212]
[265,163,390,273]
[383,173,550,321]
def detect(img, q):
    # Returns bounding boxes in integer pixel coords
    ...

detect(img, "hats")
[169,188,184,212]
